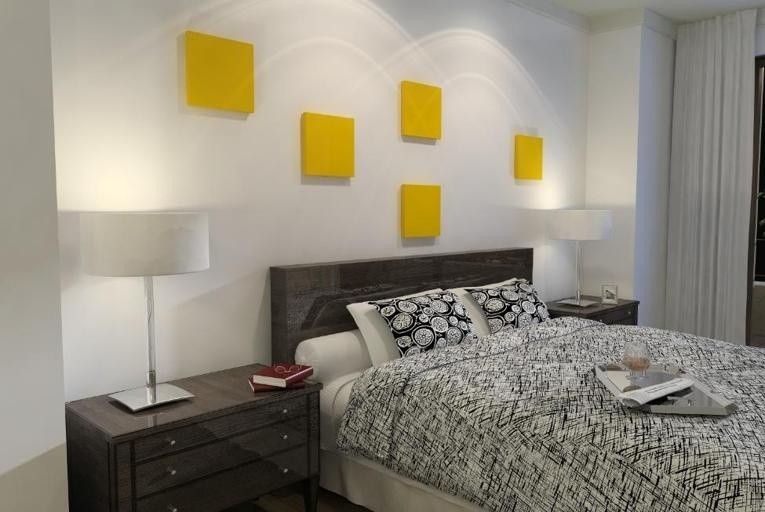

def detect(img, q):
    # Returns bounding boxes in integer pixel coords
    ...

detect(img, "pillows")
[294,277,549,382]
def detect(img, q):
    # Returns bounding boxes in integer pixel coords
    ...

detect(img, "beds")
[269,247,765,512]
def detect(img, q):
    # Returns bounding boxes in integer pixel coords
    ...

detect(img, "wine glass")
[620,341,650,381]
[621,376,640,392]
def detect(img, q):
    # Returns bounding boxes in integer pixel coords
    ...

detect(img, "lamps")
[549,209,613,309]
[78,210,212,413]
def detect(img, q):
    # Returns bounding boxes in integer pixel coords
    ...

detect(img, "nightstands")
[545,294,640,327]
[63,364,323,511]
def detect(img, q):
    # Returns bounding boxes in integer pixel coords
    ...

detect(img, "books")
[594,359,694,410]
[252,360,314,389]
[247,376,306,393]
